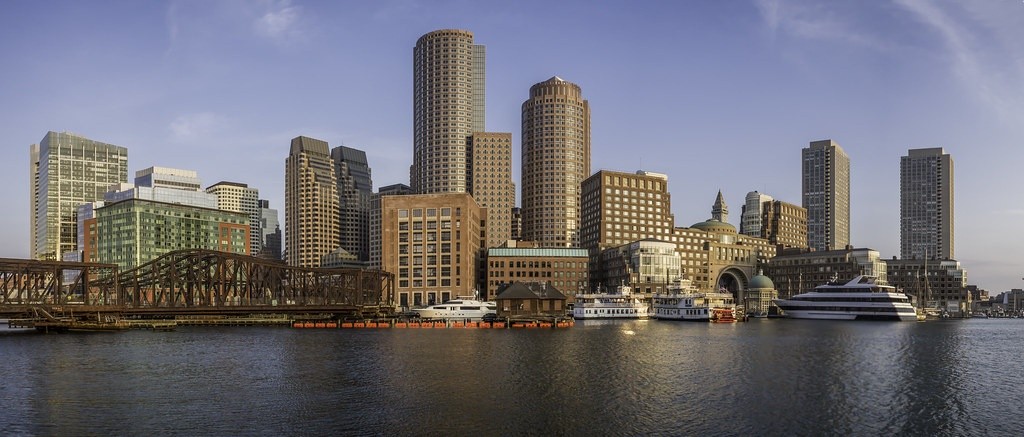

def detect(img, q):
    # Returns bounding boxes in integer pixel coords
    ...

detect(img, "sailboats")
[911,247,945,321]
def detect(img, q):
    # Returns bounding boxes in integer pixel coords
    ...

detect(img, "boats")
[411,291,508,320]
[973,313,988,318]
[771,274,918,322]
[653,277,736,320]
[573,280,655,319]
[710,307,738,322]
[943,311,950,318]
[754,315,767,317]
